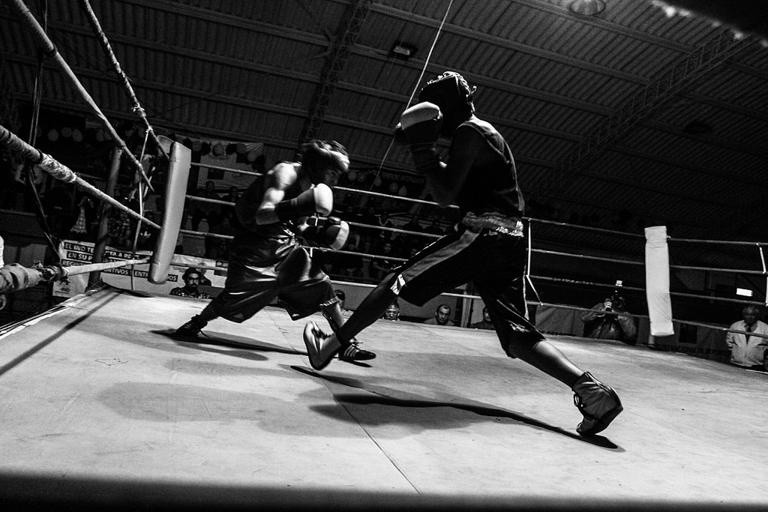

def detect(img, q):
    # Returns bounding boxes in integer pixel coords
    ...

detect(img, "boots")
[571,371,623,435]
[305,321,345,370]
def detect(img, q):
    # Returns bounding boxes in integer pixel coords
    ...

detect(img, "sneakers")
[339,342,375,361]
[175,315,206,339]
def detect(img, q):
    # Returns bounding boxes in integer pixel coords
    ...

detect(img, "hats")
[181,267,201,280]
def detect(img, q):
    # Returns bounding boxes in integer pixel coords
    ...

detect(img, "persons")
[726,307,768,371]
[580,289,637,344]
[303,70,624,437]
[1,183,498,328]
[174,139,376,361]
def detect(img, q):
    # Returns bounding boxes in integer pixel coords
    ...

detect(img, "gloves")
[395,101,444,173]
[303,217,350,250]
[277,182,334,223]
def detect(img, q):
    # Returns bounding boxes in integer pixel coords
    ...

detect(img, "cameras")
[612,280,625,312]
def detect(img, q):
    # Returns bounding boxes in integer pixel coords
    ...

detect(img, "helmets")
[303,142,348,183]
[417,71,473,119]
